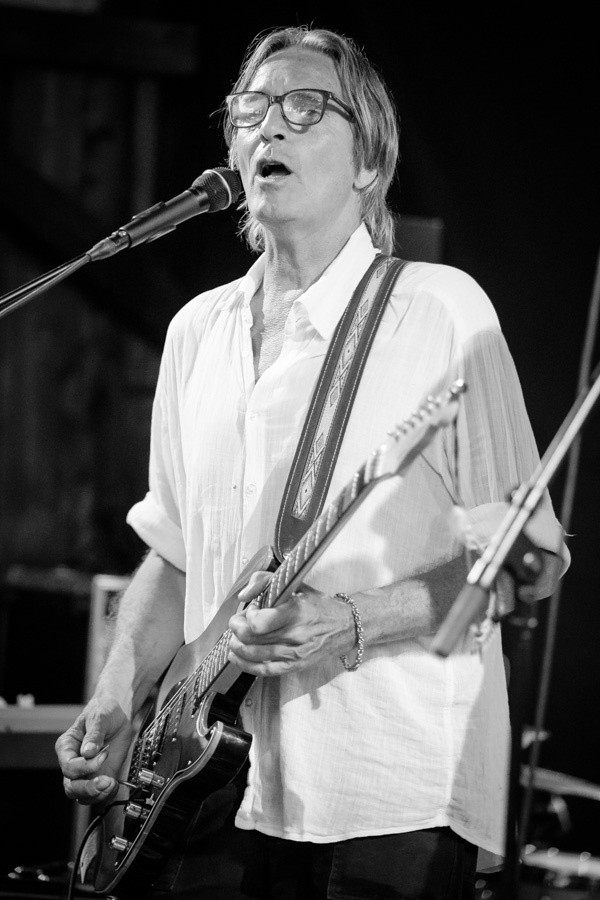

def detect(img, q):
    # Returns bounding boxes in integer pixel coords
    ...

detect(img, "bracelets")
[334,594,365,673]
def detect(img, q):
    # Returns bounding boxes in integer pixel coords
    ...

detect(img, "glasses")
[225,89,359,139]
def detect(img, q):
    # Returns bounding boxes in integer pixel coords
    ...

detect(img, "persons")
[57,26,570,900]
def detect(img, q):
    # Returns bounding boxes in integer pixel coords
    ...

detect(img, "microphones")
[85,162,245,267]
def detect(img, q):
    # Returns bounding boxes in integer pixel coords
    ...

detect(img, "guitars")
[90,378,469,899]
